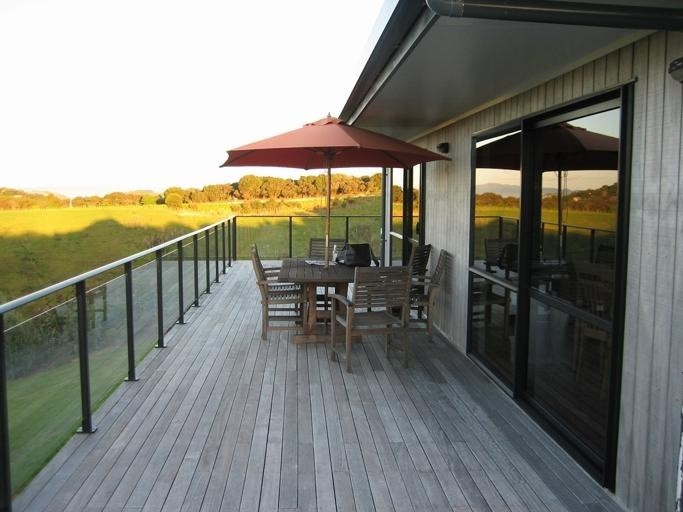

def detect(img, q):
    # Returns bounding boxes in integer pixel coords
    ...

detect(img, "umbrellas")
[216,111,454,269]
[470,116,622,264]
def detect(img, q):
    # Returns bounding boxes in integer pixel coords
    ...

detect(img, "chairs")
[250,238,447,373]
[471,239,613,383]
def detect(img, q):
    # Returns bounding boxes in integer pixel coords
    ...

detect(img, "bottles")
[332,245,338,261]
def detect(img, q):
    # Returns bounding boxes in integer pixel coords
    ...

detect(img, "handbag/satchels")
[336,243,380,267]
[499,244,519,270]
